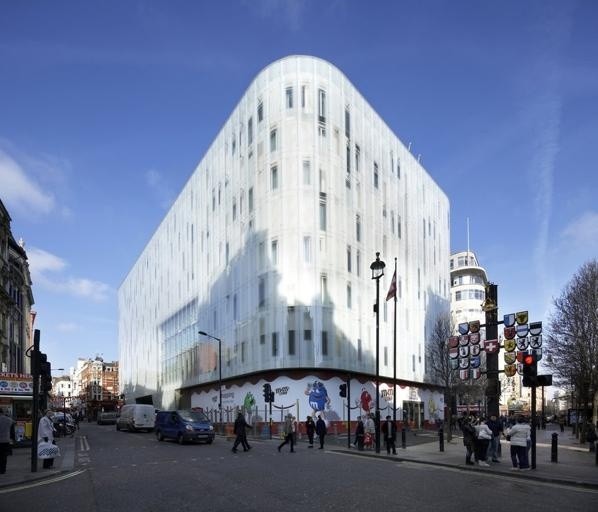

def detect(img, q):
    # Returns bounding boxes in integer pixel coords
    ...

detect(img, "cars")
[546,414,556,423]
[51,410,76,436]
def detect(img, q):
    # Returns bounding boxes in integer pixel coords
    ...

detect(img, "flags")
[386,270,396,303]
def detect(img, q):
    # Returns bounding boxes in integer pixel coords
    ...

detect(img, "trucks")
[97,401,119,425]
[114,404,156,431]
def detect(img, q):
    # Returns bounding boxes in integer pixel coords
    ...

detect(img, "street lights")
[51,367,65,371]
[370,249,385,453]
[198,330,223,434]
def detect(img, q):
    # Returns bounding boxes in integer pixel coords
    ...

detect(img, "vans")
[155,408,217,444]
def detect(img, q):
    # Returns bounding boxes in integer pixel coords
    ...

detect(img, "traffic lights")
[262,383,271,401]
[43,362,52,391]
[523,354,536,386]
[339,383,346,398]
[32,351,47,376]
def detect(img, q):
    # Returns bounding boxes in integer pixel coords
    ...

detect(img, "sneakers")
[509,466,531,472]
[466,458,501,468]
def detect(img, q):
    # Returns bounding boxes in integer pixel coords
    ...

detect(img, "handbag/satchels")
[37,442,62,460]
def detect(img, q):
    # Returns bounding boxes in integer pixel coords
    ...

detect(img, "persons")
[0,406,17,474]
[582,418,597,452]
[455,408,532,471]
[231,409,253,453]
[233,412,253,451]
[37,407,58,469]
[305,416,316,447]
[277,413,296,453]
[381,414,398,455]
[51,405,89,437]
[315,414,328,449]
[290,416,298,445]
[348,412,376,451]
[559,414,565,432]
